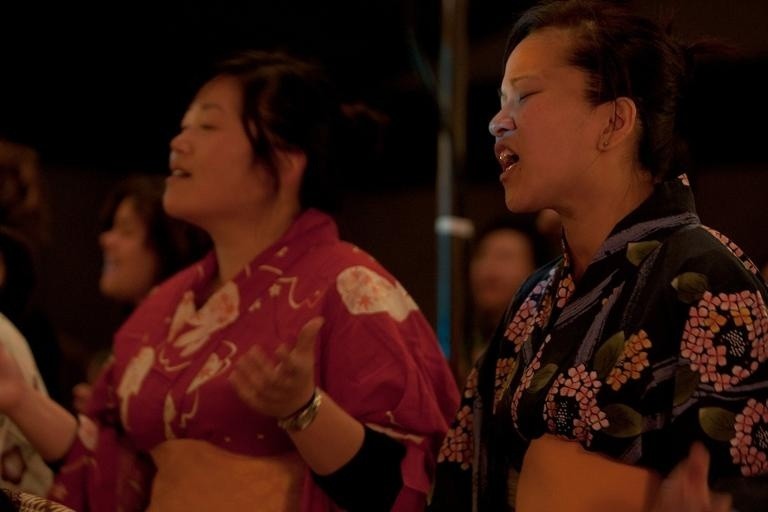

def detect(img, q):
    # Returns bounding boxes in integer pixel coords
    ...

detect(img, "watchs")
[279,386,323,434]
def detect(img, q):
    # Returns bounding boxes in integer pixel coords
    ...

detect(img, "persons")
[431,2,768,510]
[0,144,84,422]
[0,45,463,512]
[456,214,539,379]
[95,177,205,309]
